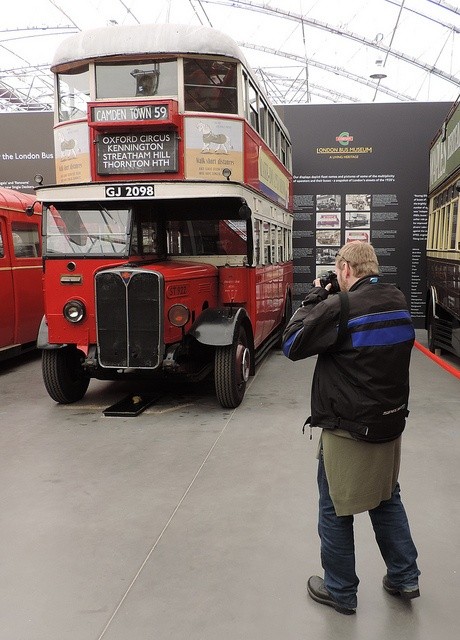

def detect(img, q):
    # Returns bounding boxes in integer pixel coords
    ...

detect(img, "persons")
[281,241,422,614]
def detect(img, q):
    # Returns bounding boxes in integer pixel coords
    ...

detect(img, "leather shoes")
[383,575,420,599]
[307,575,356,615]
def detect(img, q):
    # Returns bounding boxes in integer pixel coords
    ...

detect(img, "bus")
[0,185,66,352]
[37,23,293,409]
[423,93,460,358]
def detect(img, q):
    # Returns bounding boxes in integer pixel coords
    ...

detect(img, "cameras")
[312,270,341,294]
